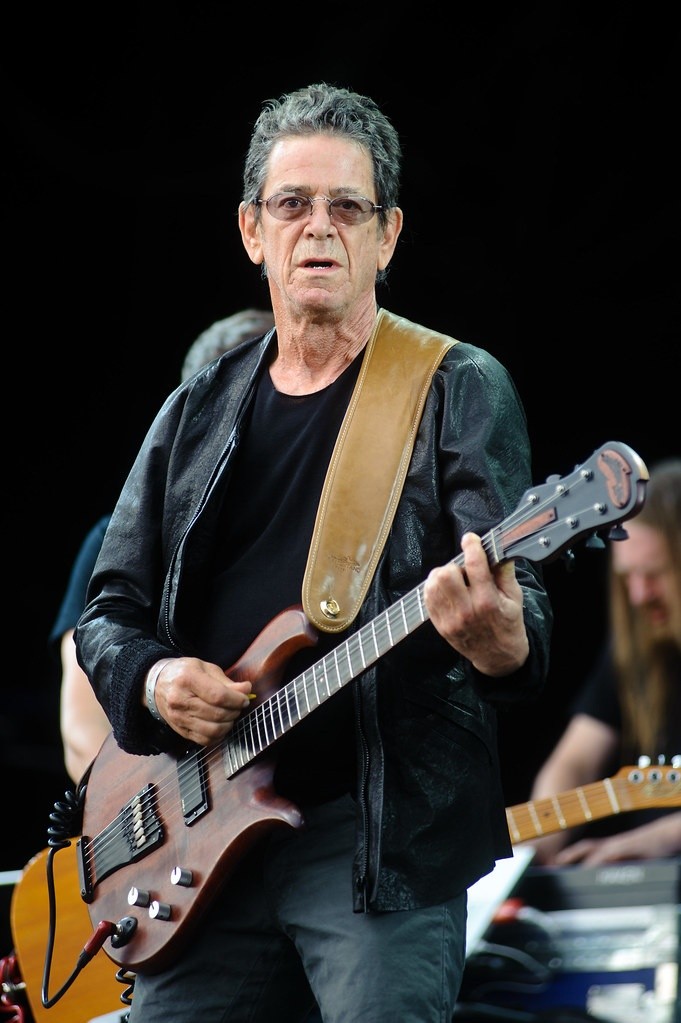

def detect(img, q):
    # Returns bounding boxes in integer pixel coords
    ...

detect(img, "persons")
[52,312,274,785]
[525,458,681,871]
[73,82,556,1023]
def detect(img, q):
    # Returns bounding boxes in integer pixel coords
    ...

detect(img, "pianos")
[504,849,681,914]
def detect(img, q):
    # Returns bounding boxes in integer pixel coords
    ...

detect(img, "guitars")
[10,747,680,1023]
[70,439,650,978]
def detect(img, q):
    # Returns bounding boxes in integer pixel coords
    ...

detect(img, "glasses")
[252,192,389,226]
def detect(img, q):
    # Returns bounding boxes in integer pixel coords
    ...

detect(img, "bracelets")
[146,657,176,725]
